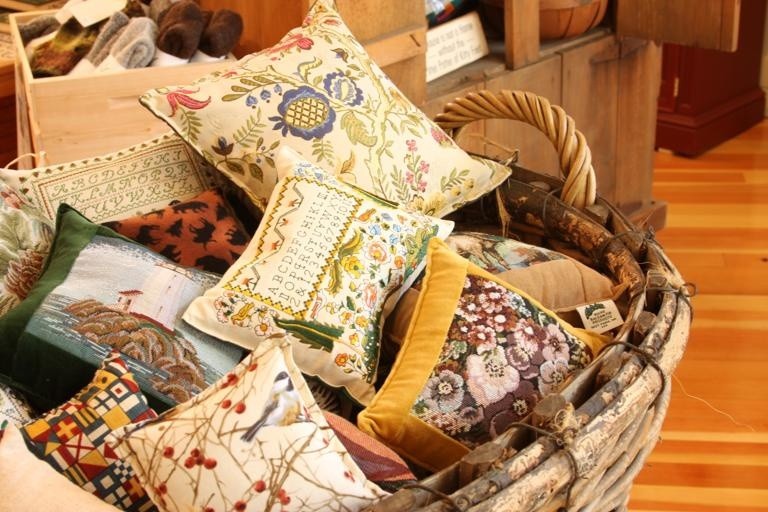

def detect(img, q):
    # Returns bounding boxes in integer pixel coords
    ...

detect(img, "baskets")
[357,89,695,511]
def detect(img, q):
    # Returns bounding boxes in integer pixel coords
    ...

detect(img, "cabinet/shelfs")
[380,35,663,222]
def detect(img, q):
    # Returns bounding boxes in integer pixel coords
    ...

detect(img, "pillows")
[178,147,457,404]
[355,234,609,474]
[3,202,246,414]
[20,346,161,511]
[103,327,393,512]
[134,2,513,226]
